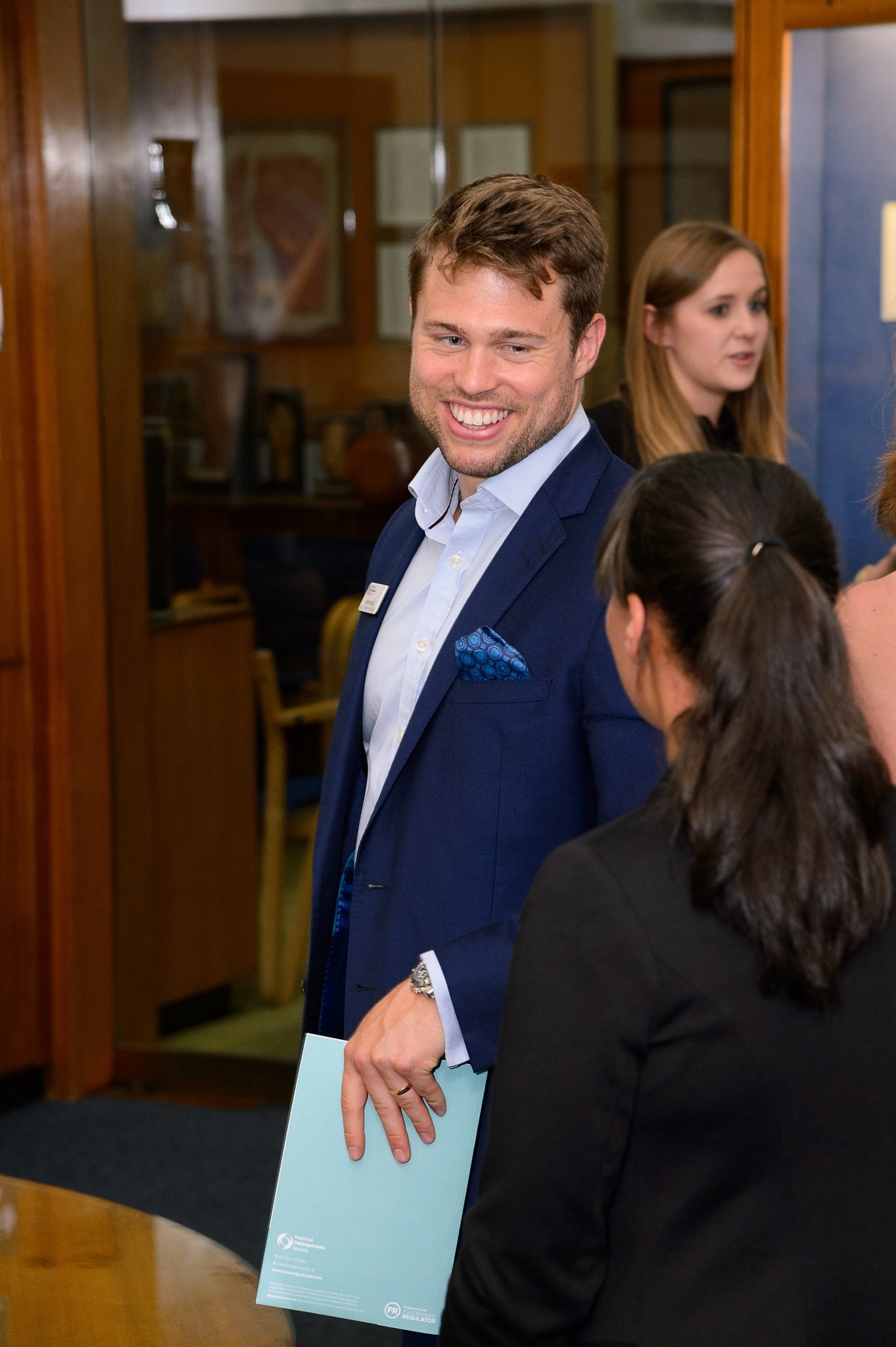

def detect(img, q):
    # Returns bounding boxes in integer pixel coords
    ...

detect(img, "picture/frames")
[221,110,343,348]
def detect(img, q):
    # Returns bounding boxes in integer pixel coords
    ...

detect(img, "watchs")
[409,960,437,1002]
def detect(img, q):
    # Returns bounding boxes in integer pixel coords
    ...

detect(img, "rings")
[388,1083,412,1095]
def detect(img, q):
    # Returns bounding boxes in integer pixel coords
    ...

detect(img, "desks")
[0,1175,297,1347]
[146,608,256,1039]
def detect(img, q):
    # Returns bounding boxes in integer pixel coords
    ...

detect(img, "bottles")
[349,409,414,508]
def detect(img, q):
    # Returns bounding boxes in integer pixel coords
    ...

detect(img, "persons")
[584,222,791,475]
[298,172,672,1347]
[438,444,896,1347]
